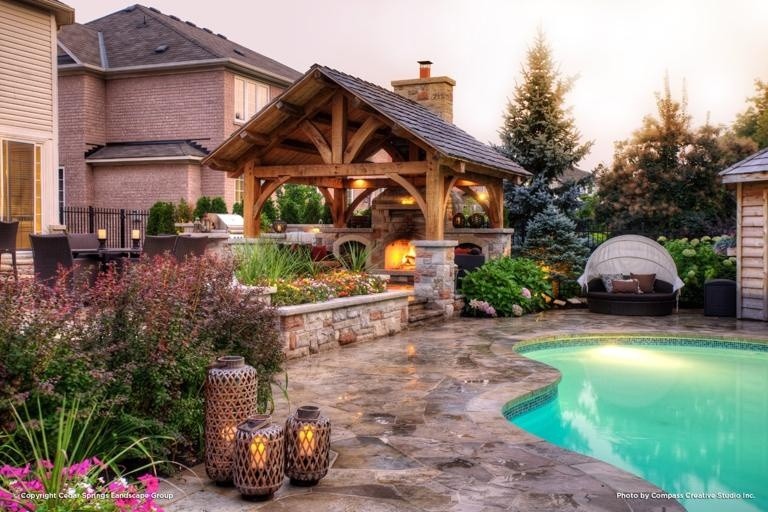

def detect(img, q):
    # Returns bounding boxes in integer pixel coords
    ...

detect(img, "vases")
[273,221,288,235]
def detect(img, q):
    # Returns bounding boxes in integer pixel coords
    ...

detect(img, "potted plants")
[224,232,281,308]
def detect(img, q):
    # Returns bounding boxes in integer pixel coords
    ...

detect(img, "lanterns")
[282,403,332,488]
[234,413,286,501]
[204,355,260,489]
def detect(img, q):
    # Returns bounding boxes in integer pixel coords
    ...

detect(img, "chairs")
[27,234,72,287]
[0,220,20,285]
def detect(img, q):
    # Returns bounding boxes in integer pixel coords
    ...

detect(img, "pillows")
[598,273,625,292]
[611,280,639,293]
[630,272,656,294]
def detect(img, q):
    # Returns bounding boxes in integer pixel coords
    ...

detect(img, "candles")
[132,229,140,239]
[98,229,106,239]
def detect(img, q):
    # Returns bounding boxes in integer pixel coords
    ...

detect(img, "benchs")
[66,233,100,259]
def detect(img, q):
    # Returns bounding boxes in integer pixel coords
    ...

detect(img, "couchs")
[587,275,676,317]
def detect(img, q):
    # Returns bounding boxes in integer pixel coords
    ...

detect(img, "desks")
[71,248,143,265]
